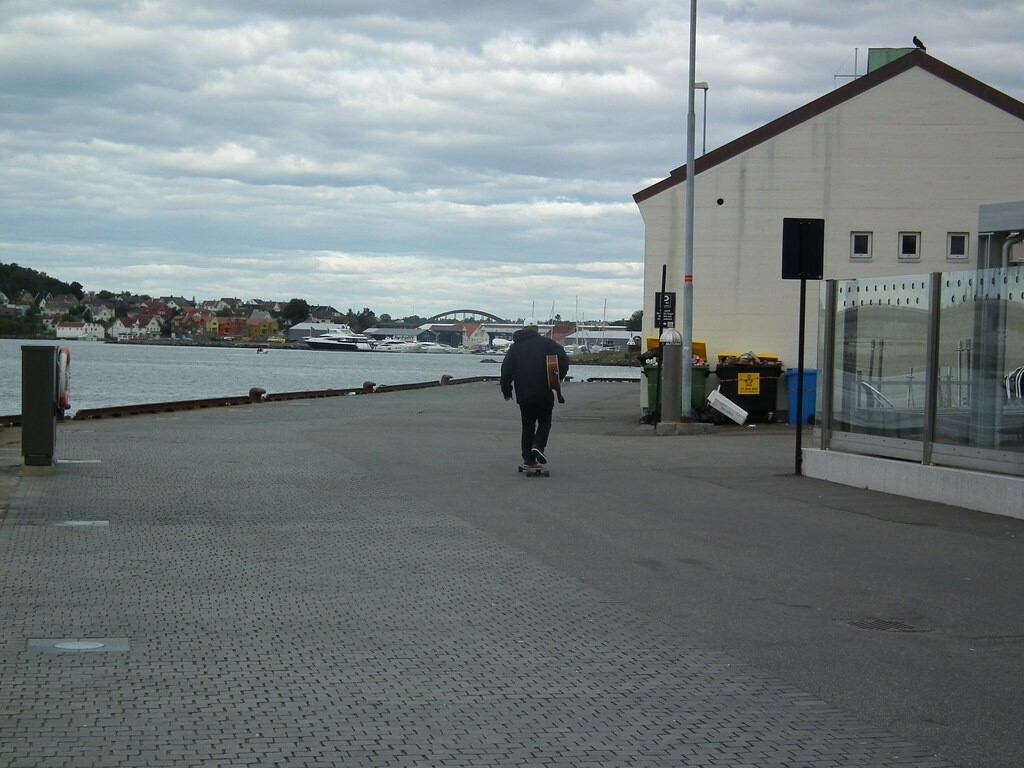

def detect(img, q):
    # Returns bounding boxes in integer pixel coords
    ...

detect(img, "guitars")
[546,353,562,391]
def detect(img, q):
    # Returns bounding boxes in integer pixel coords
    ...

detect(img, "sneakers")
[531,445,547,464]
[522,460,538,467]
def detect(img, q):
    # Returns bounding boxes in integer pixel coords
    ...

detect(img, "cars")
[220,333,235,342]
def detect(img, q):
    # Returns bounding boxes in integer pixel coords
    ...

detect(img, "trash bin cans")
[786,367,820,427]
[713,353,782,425]
[645,336,710,412]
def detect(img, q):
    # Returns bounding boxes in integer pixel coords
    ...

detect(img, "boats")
[257,351,268,354]
[373,339,418,352]
[304,328,379,352]
[415,338,622,354]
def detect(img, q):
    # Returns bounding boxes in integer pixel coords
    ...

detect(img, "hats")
[524,318,537,326]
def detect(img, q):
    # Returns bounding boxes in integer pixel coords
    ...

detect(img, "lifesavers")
[56,345,74,414]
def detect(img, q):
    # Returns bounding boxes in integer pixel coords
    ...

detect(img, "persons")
[500,318,569,464]
